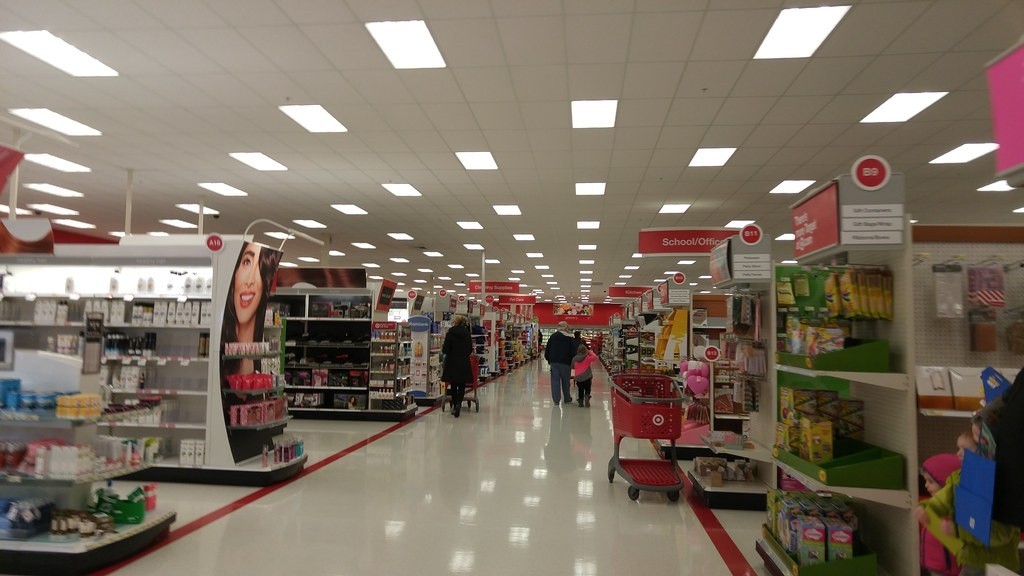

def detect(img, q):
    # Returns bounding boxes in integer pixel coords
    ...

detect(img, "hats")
[559,321,567,327]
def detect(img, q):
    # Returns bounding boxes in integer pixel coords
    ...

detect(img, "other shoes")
[452,401,461,417]
[564,398,572,403]
[554,401,559,405]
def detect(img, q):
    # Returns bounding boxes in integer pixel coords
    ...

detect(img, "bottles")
[198,332,209,356]
[104,333,154,356]
[378,389,395,400]
[380,330,396,371]
[430,336,441,349]
[262,435,304,468]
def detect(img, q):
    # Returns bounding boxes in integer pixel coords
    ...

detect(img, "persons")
[538,330,542,352]
[221,242,284,375]
[442,315,473,418]
[543,321,597,408]
[912,411,1021,576]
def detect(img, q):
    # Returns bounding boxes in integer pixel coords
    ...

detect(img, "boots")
[577,399,583,407]
[584,394,591,407]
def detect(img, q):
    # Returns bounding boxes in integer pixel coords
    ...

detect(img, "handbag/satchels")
[954,426,995,548]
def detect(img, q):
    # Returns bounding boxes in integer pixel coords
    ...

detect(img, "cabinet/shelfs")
[596,223,1024,576]
[0,255,543,576]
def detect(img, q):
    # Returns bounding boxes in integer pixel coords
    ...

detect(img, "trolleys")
[442,354,479,412]
[608,369,685,502]
